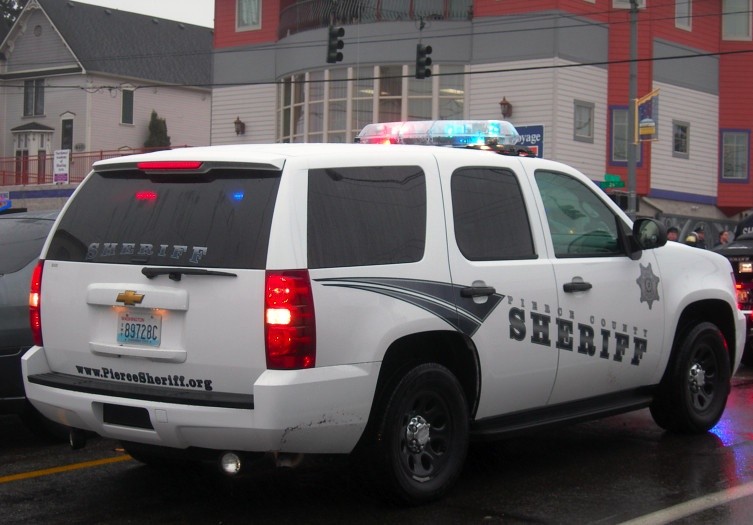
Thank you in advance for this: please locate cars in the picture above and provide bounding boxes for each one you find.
[0,186,102,446]
[712,214,753,332]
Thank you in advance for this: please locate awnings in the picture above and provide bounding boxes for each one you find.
[639,196,727,222]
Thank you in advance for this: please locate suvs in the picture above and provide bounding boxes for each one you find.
[20,118,753,506]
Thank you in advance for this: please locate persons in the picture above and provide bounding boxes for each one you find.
[668,227,729,250]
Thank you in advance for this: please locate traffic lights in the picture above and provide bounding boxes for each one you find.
[415,43,433,81]
[325,26,346,65]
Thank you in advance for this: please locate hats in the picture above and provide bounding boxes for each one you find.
[695,227,705,233]
